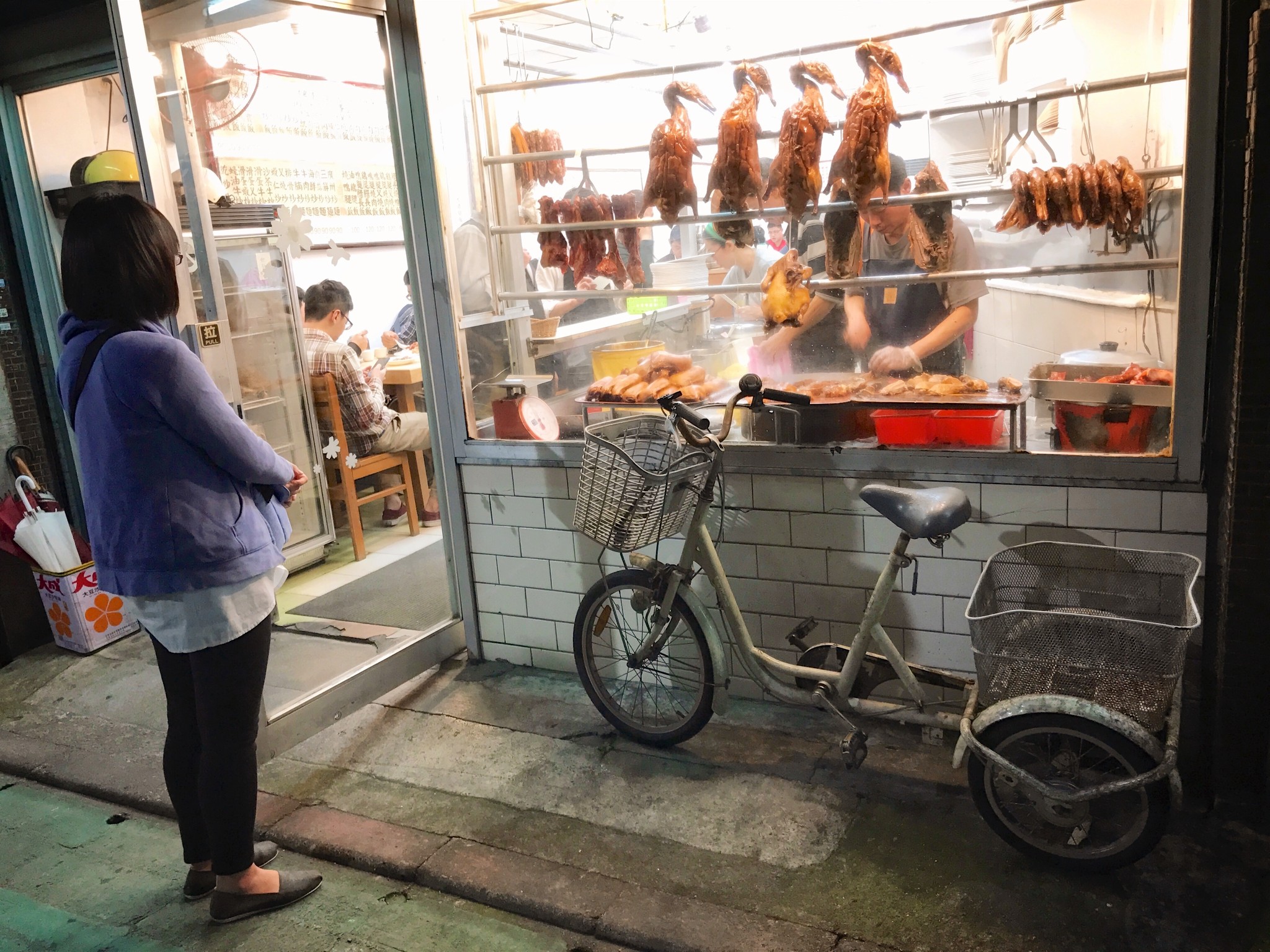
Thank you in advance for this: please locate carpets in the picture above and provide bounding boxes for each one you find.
[285,539,451,631]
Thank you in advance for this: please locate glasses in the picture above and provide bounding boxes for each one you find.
[174,252,182,266]
[406,294,413,301]
[332,309,353,330]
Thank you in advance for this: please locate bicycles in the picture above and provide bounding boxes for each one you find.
[569,376,1203,873]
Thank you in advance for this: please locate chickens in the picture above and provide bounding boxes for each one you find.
[761,249,813,334]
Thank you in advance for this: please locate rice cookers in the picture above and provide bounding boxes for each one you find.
[1040,343,1164,367]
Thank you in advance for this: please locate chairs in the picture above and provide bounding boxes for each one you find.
[311,373,419,561]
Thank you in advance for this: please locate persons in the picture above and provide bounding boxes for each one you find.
[380,267,420,352]
[301,277,454,526]
[291,285,368,358]
[837,150,991,375]
[751,226,774,250]
[700,222,790,320]
[54,191,323,922]
[535,189,635,325]
[738,157,858,372]
[765,222,789,254]
[449,194,532,378]
[607,189,655,313]
[657,224,700,262]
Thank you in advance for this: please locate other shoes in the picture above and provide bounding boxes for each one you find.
[208,868,322,922]
[183,841,280,900]
[423,505,442,527]
[382,503,408,526]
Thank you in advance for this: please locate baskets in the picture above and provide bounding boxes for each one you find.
[964,538,1201,734]
[573,414,714,553]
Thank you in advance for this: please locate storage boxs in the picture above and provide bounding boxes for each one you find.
[31,560,141,657]
[626,296,667,314]
[870,408,1004,445]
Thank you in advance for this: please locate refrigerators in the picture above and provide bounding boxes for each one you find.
[181,204,336,576]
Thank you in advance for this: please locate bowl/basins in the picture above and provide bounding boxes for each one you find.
[680,336,738,366]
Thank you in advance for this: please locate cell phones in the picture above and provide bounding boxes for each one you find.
[368,357,390,376]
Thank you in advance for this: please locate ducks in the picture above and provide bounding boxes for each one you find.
[994,155,1145,236]
[822,40,910,211]
[638,81,717,229]
[762,60,848,222]
[704,62,776,214]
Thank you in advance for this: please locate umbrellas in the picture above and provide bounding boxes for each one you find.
[9,474,82,572]
[0,490,47,568]
[5,443,91,567]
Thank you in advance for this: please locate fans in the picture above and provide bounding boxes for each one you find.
[154,30,260,182]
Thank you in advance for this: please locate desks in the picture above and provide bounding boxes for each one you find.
[362,354,433,517]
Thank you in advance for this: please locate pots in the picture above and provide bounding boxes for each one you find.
[589,341,665,383]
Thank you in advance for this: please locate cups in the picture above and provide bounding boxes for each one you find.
[375,348,387,358]
[249,424,266,441]
[362,349,375,361]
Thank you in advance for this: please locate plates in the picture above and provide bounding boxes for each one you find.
[386,360,413,365]
[394,350,412,356]
[649,252,716,303]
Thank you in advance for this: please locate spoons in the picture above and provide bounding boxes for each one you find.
[395,339,410,349]
